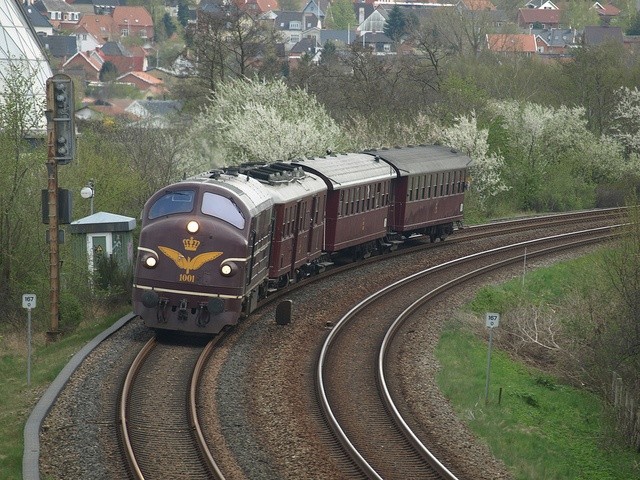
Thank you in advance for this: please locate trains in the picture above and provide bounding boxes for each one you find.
[127,140,473,334]
